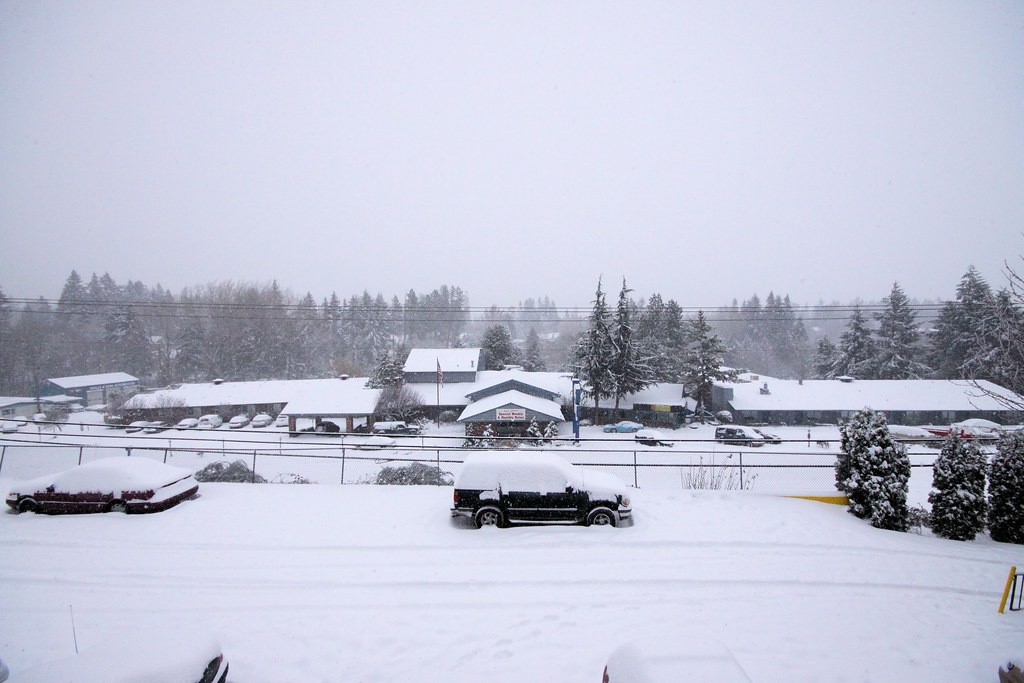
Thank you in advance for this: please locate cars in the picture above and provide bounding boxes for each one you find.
[14,416,28,426]
[228,415,251,429]
[753,427,782,444]
[884,418,1002,447]
[6,458,202,516]
[276,414,289,427]
[143,420,170,433]
[2,420,19,433]
[175,418,199,432]
[252,413,275,429]
[70,404,85,413]
[30,413,47,423]
[126,420,149,433]
[602,421,643,434]
[635,429,675,447]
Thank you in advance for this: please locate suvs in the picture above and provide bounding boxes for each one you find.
[315,421,341,434]
[371,422,421,437]
[450,451,635,529]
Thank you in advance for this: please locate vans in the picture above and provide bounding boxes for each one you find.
[715,426,766,447]
[199,414,223,430]
